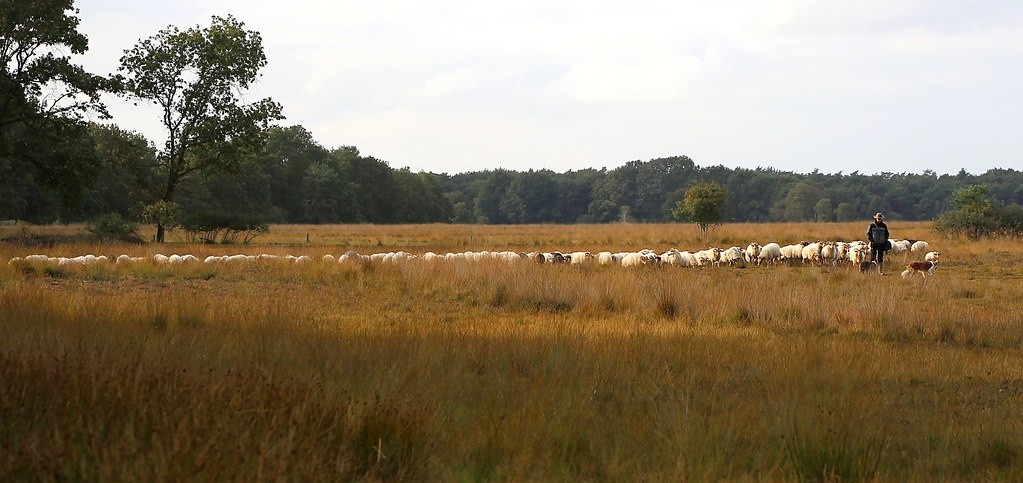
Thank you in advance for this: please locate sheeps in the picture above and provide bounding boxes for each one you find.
[7,237,942,271]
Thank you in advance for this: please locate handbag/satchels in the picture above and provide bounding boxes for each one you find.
[883,240,891,251]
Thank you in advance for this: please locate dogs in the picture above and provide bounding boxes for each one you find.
[901,258,936,279]
[859,258,878,276]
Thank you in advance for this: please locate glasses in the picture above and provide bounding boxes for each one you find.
[878,219,881,220]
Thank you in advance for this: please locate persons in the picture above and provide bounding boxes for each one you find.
[866,213,890,276]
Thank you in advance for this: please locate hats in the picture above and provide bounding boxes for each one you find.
[873,213,884,219]
